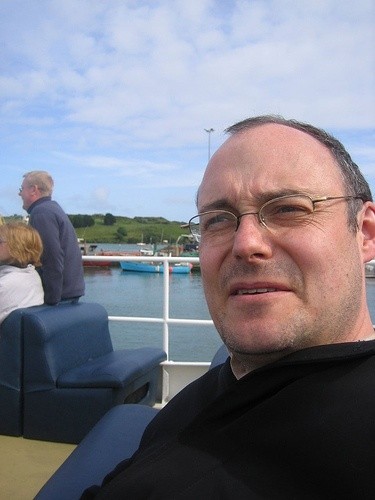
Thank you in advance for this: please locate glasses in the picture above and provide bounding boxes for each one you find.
[177,194,367,248]
[19,185,34,192]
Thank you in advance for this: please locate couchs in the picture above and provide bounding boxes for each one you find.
[0,302,167,446]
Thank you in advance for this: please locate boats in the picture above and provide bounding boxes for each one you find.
[77,230,201,275]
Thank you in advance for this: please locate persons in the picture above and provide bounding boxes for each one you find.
[0,216,45,326]
[19,170,86,307]
[78,115,375,500]
